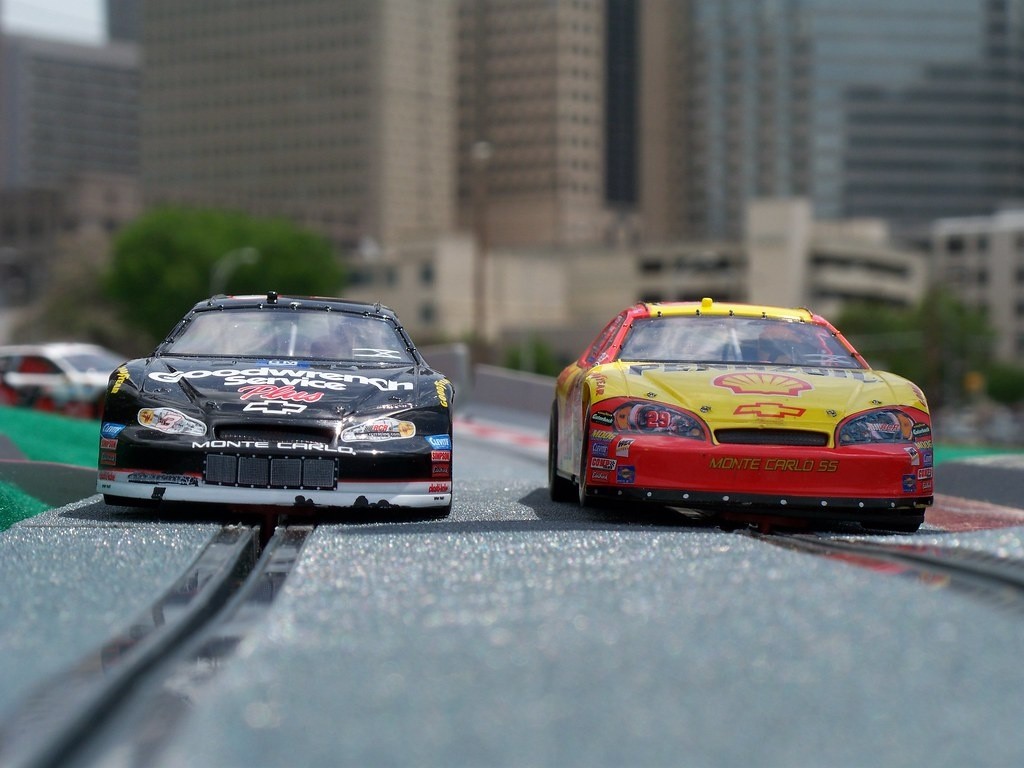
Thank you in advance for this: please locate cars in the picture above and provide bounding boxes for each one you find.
[0,343,132,419]
[549,298,934,533]
[95,291,455,516]
